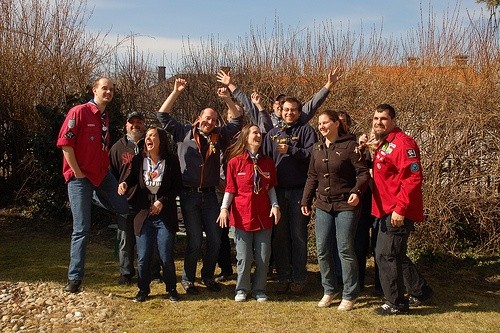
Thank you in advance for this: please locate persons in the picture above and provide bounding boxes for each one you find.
[57,77,129,289]
[215,65,346,280]
[117,126,181,304]
[321,112,371,291]
[358,122,404,299]
[263,97,320,296]
[215,123,281,302]
[206,92,277,284]
[156,78,247,295]
[302,110,372,312]
[356,103,435,314]
[106,111,165,287]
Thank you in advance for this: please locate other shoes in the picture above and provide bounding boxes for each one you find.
[153,274,164,283]
[118,271,136,284]
[202,275,220,291]
[214,274,236,282]
[64,280,82,293]
[181,280,200,296]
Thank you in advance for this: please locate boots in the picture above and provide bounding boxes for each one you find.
[289,276,309,294]
[269,278,293,293]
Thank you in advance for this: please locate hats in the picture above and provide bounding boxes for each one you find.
[127,112,145,122]
[275,93,288,101]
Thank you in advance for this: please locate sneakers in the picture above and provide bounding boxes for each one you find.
[337,294,359,311]
[168,291,179,303]
[132,290,150,303]
[251,292,269,302]
[318,291,341,308]
[374,304,400,315]
[407,287,432,306]
[235,289,249,302]
